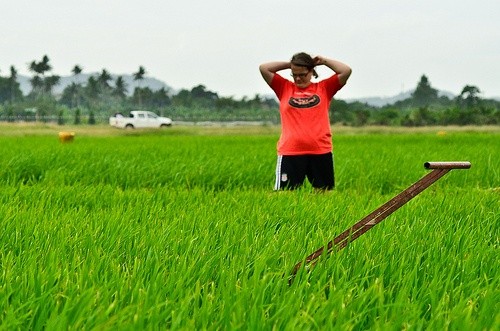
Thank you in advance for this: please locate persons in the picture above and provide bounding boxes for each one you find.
[259,52,353,193]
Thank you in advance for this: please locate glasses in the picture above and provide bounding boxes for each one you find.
[290,71,310,78]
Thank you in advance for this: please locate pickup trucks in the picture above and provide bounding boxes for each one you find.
[108,111,172,130]
